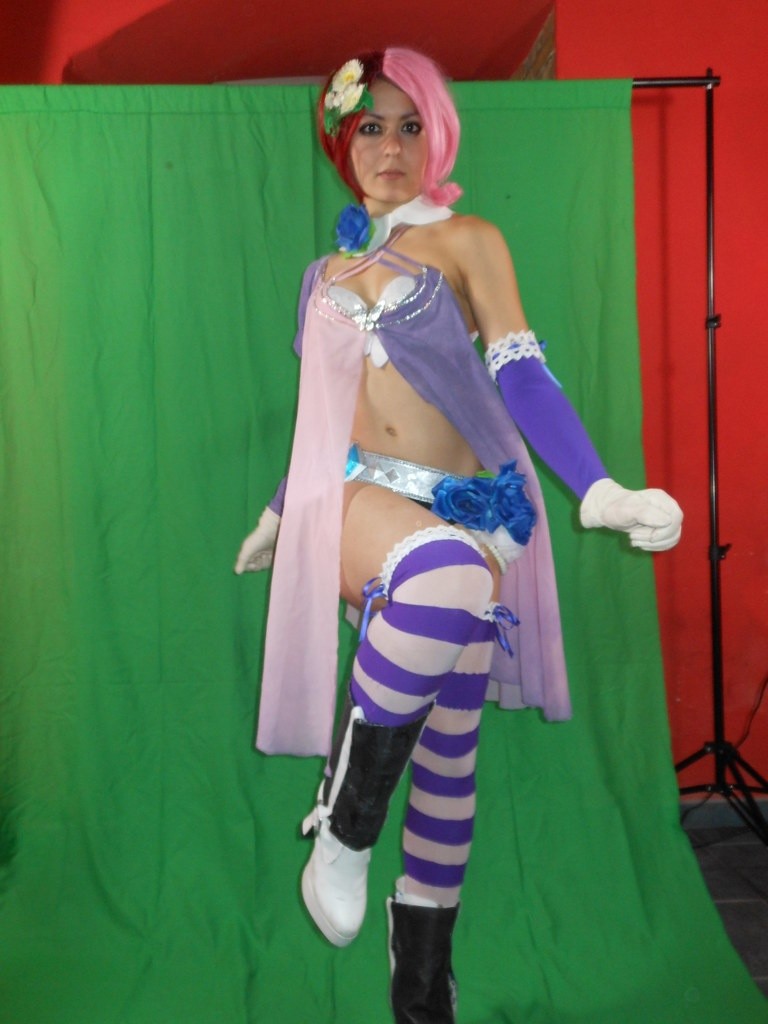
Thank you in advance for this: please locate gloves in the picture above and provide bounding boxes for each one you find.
[234,507,281,574]
[579,476,684,552]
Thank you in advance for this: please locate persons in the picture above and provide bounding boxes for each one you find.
[233,50,685,1023]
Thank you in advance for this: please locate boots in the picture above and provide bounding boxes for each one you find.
[300,681,436,947]
[383,895,459,1024]
